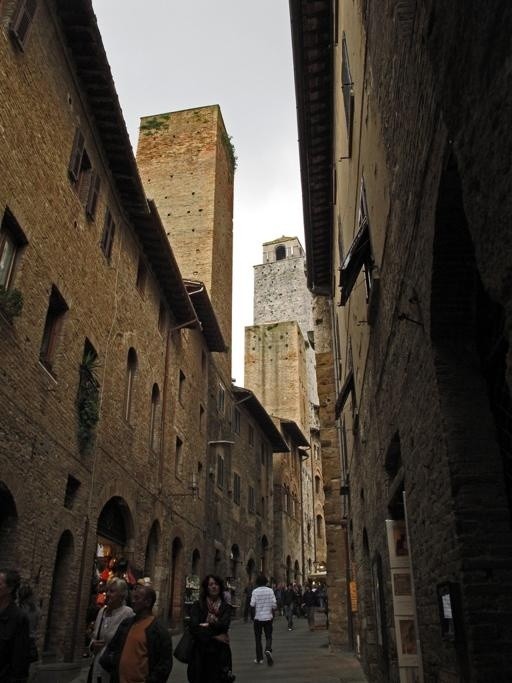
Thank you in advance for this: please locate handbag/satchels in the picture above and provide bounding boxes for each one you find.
[86,655,95,683]
[172,624,200,664]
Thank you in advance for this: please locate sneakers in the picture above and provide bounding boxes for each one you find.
[253,657,265,664]
[265,650,274,665]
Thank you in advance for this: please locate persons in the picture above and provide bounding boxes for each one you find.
[100,586,171,683]
[14,578,40,627]
[220,581,331,633]
[2,561,33,682]
[86,575,135,682]
[188,575,236,682]
[248,575,278,668]
